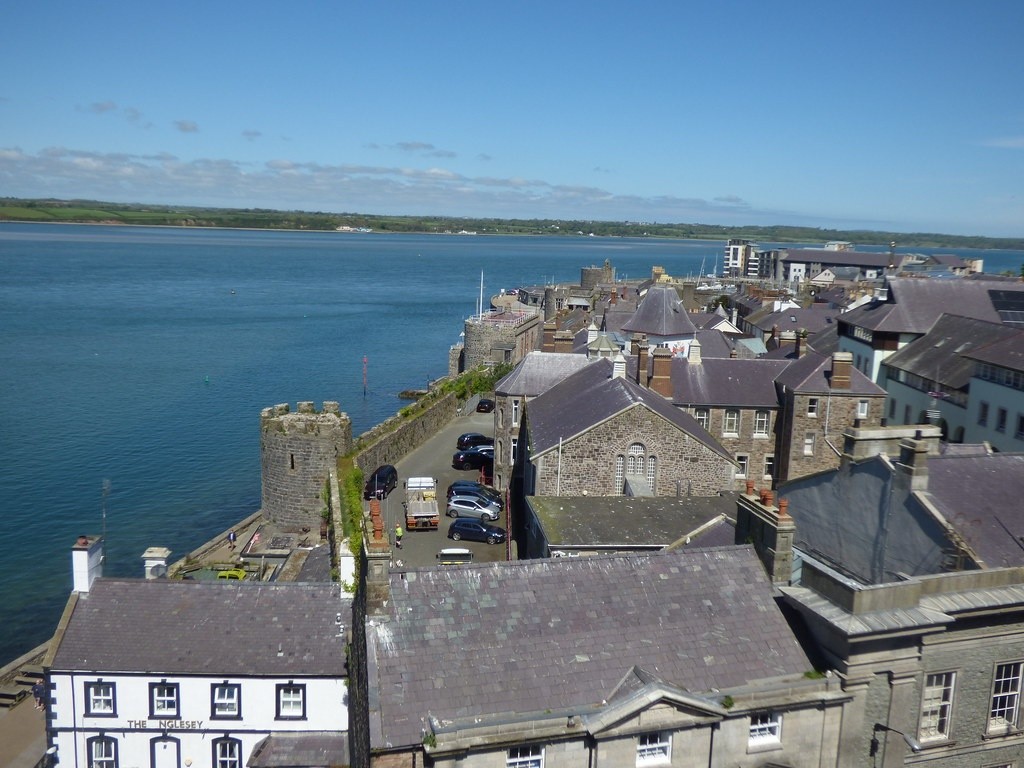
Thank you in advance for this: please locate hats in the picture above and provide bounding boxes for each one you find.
[397,524,399,526]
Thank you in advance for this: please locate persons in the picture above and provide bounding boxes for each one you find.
[228,530,236,551]
[31,679,46,709]
[395,524,403,549]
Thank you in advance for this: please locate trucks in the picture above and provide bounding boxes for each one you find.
[402,477,439,530]
[436,548,474,565]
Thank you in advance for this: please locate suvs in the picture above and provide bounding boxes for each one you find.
[457,432,494,448]
[453,449,493,471]
[363,465,398,500]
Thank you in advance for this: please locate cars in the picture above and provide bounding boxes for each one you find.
[477,398,494,413]
[448,517,508,545]
[445,481,504,512]
[446,494,501,522]
[470,444,494,459]
